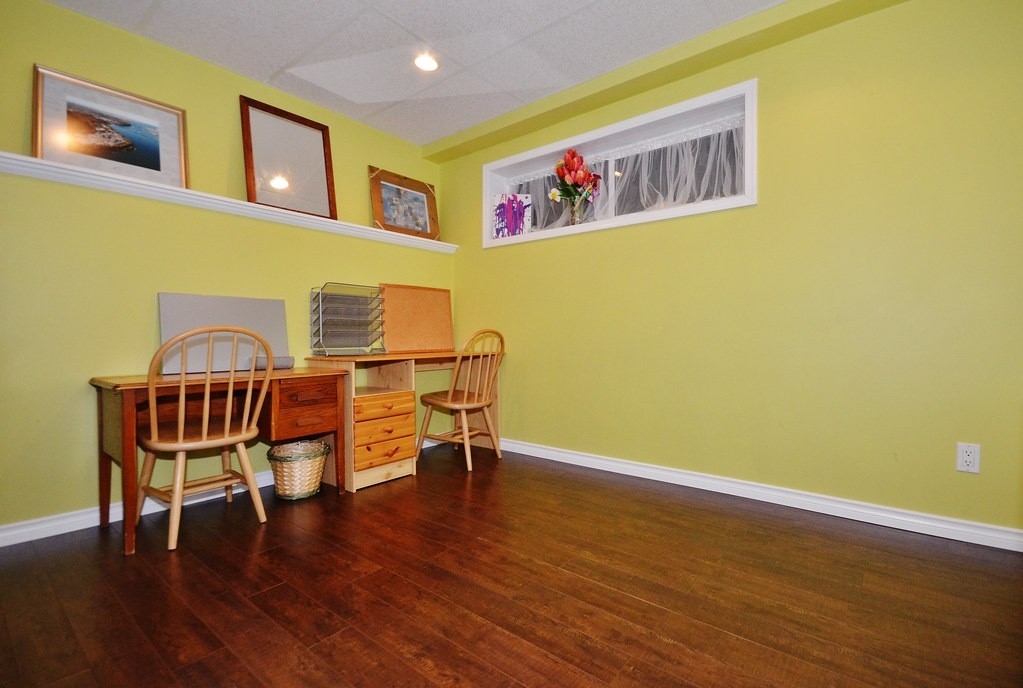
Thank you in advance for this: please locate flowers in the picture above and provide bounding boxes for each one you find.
[547,148,602,210]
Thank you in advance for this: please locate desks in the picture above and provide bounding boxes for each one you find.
[88,366,351,557]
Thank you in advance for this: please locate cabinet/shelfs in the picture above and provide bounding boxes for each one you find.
[303,352,506,496]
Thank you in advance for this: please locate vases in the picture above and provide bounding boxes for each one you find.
[568,195,583,226]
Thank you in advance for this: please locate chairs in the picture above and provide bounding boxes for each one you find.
[136,325,274,552]
[415,329,508,472]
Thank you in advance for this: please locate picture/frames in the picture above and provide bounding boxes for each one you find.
[368,164,441,242]
[31,62,190,189]
[239,94,338,221]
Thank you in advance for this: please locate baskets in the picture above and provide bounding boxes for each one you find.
[267,439,331,500]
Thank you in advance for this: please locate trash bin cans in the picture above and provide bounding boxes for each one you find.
[266,439,330,501]
